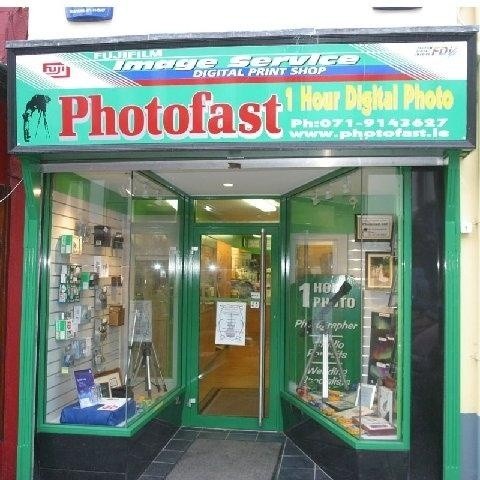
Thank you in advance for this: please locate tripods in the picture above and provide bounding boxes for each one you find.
[133,350,160,397]
[297,333,349,403]
